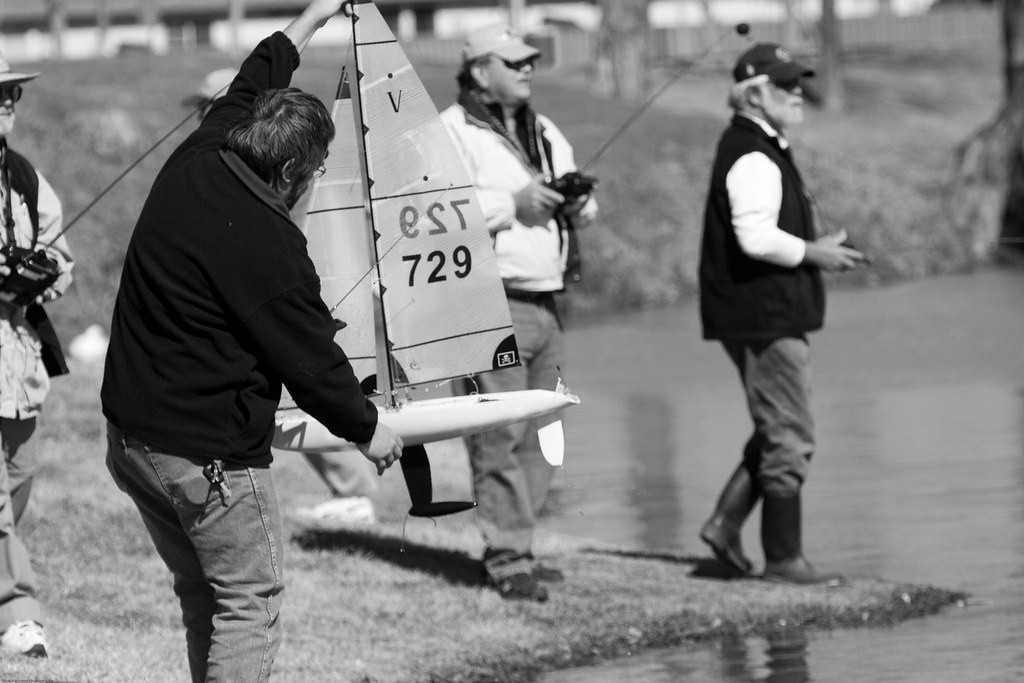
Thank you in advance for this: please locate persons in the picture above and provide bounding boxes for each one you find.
[436,22,599,600]
[700,44,864,583]
[1,61,74,658]
[100,0,404,682]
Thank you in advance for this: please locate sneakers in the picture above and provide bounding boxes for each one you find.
[0,620,51,657]
[482,547,564,603]
[294,495,376,528]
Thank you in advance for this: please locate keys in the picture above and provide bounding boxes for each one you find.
[203,459,232,498]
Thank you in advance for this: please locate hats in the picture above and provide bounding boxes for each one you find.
[732,42,816,81]
[183,66,239,101]
[0,59,41,83]
[459,21,543,64]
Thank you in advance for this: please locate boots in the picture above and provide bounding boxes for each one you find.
[700,458,761,573]
[761,489,846,587]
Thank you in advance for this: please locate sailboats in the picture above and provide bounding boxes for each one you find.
[268,0,578,474]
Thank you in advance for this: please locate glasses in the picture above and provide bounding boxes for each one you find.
[306,161,327,179]
[0,83,23,104]
